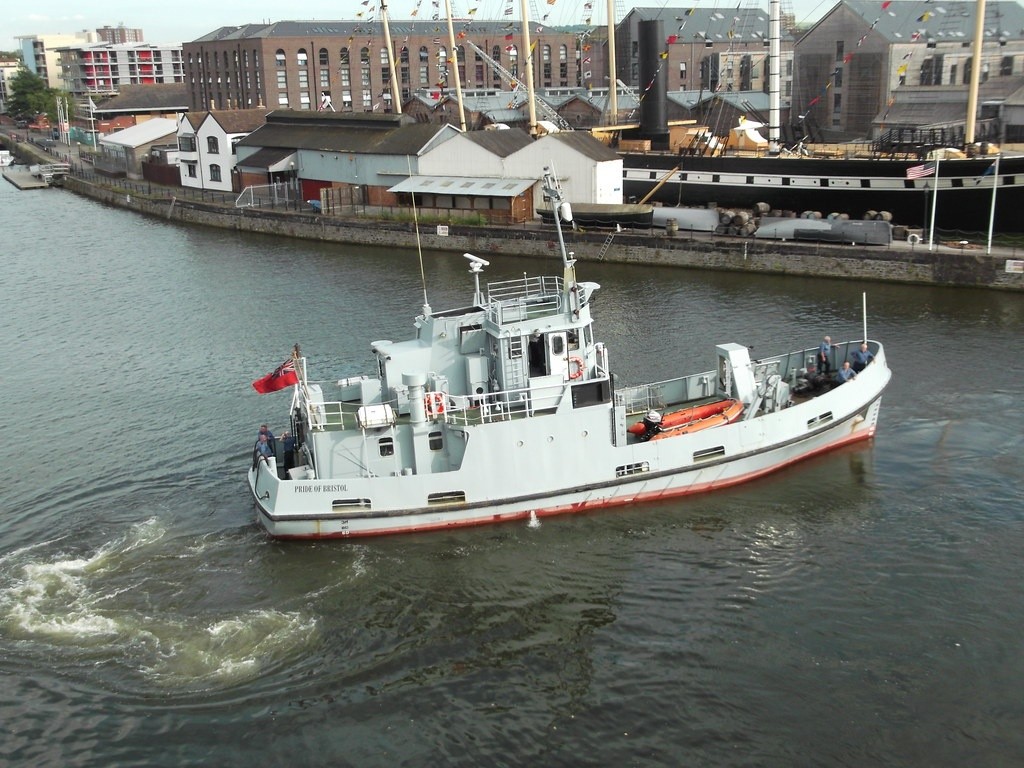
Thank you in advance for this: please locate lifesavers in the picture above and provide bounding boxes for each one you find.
[423,393,445,415]
[567,356,586,380]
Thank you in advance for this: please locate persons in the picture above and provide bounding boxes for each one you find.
[279,430,295,480]
[830,362,856,390]
[817,335,840,376]
[850,343,876,374]
[256,425,276,458]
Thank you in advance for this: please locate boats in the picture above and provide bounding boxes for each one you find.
[248,168,890,543]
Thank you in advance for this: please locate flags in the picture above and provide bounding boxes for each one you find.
[505,44,513,52]
[582,44,591,50]
[253,357,299,393]
[976,159,996,185]
[505,6,513,16]
[584,70,591,79]
[582,56,591,63]
[906,160,936,179]
[506,33,513,40]
